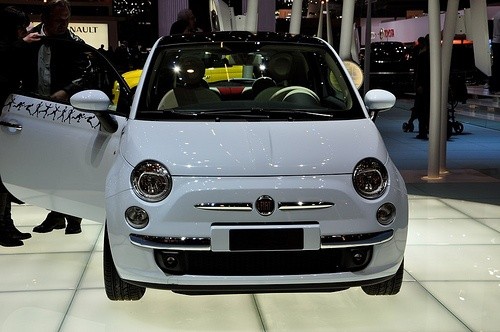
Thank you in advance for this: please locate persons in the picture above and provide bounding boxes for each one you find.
[0,5,42,247]
[413,39,430,140]
[21,0,97,236]
[170,9,196,36]
[98,44,106,51]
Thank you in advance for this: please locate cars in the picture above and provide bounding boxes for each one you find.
[360,40,415,69]
[0,33,408,302]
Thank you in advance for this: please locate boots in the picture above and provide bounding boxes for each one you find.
[0,224,24,247]
[6,219,31,240]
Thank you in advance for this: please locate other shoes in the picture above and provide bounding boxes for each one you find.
[33,219,65,233]
[64,223,81,234]
[416,135,427,139]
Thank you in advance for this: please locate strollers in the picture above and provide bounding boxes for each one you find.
[402,89,464,138]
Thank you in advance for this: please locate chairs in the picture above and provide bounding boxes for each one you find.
[253,84,293,102]
[157,86,221,111]
[177,56,209,90]
[267,54,298,88]
